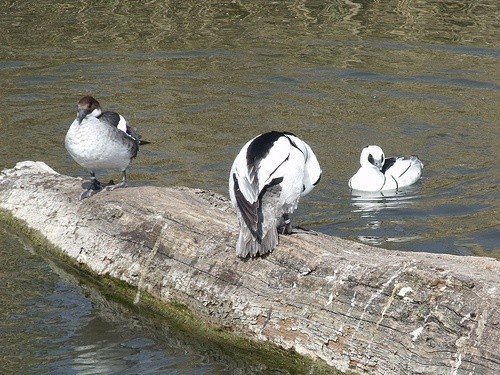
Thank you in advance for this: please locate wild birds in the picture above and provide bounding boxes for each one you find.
[71,315,140,375]
[351,189,427,246]
[228,131,323,258]
[348,145,425,192]
[64,95,155,201]
